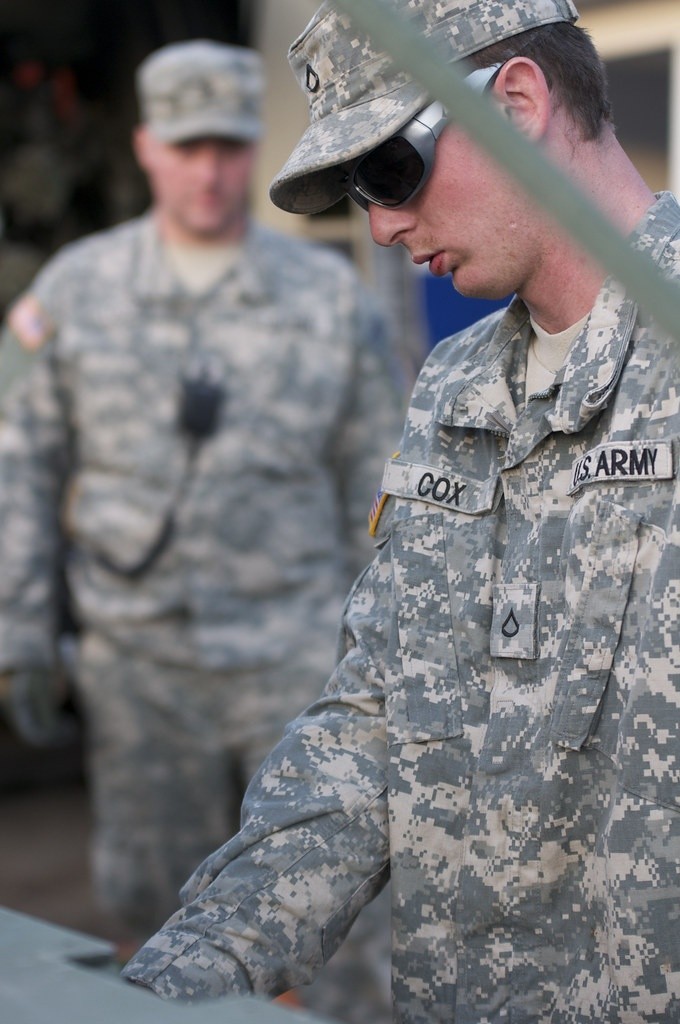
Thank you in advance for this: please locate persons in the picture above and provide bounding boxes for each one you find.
[114,0,680,1023]
[1,43,415,964]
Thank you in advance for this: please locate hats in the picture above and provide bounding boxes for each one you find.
[271,3,579,207]
[138,41,272,145]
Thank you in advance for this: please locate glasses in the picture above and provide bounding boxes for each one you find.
[342,64,502,210]
